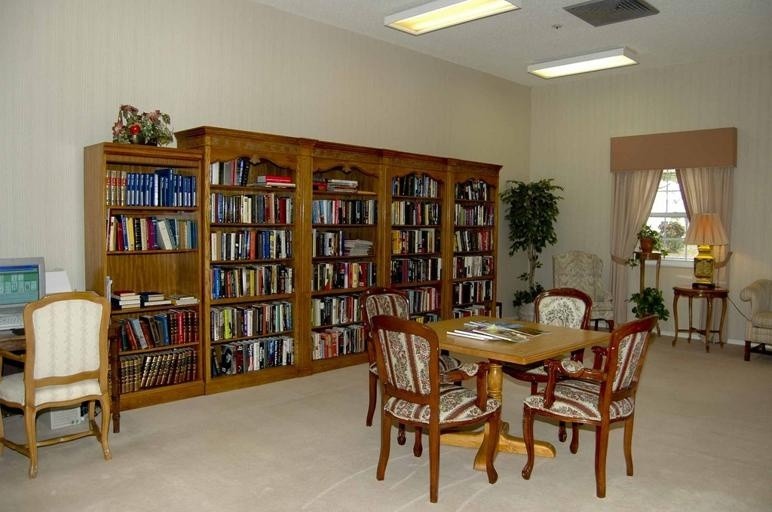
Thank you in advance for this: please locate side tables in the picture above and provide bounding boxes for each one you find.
[671,285,730,353]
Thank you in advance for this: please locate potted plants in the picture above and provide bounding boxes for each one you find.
[498,178,565,322]
[625,224,668,269]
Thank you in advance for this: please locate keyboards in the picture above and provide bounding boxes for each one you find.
[0,315,24,330]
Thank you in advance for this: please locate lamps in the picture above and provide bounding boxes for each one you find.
[383,0,522,37]
[527,46,640,81]
[683,212,728,290]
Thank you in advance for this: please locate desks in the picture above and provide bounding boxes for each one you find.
[423,315,611,473]
[0,329,27,375]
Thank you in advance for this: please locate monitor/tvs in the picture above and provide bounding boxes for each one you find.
[0,256,46,309]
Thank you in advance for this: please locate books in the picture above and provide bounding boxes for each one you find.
[107,346,199,397]
[452,254,495,279]
[312,176,372,192]
[210,192,295,225]
[210,263,295,301]
[210,300,293,342]
[209,156,297,189]
[390,175,440,198]
[389,227,442,255]
[108,310,201,354]
[106,213,199,252]
[309,293,362,328]
[390,200,441,226]
[451,280,493,305]
[446,319,552,343]
[311,260,378,291]
[105,168,198,208]
[210,228,294,262]
[312,198,377,226]
[310,323,366,361]
[403,286,441,323]
[310,228,375,258]
[451,304,494,319]
[453,181,494,253]
[106,274,199,310]
[389,256,443,286]
[210,334,295,378]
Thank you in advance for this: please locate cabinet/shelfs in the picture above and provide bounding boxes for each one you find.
[173,125,503,395]
[83,142,204,414]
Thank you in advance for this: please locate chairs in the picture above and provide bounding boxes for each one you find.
[488,287,592,442]
[359,285,463,446]
[0,290,112,479]
[552,250,616,333]
[369,315,503,504]
[522,314,659,498]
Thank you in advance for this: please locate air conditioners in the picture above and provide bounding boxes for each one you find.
[562,0,660,28]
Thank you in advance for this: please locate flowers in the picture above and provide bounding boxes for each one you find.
[112,104,174,147]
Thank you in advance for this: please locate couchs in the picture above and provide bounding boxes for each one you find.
[739,278,772,362]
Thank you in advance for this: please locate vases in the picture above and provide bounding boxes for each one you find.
[127,135,146,145]
